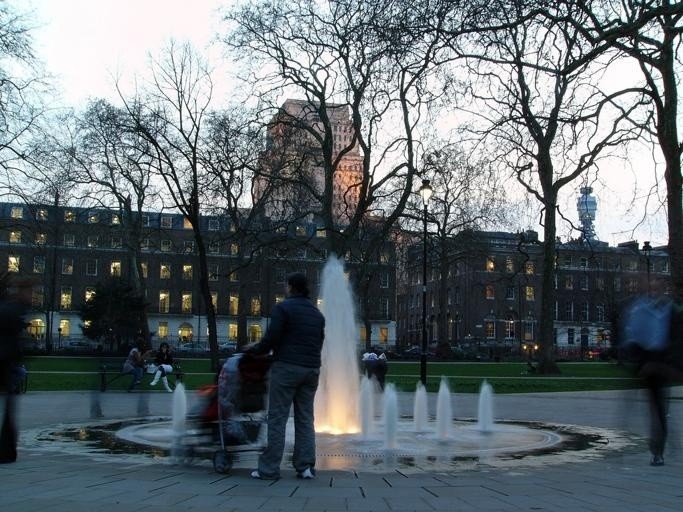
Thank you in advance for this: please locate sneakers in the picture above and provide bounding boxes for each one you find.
[294,466,315,480]
[249,468,280,482]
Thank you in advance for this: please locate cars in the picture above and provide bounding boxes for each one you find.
[377,344,603,362]
[21,334,240,357]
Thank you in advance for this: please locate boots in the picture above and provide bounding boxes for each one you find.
[149,369,162,386]
[160,375,173,393]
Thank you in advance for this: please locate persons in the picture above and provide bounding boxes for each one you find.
[121,336,155,395]
[612,265,683,468]
[148,341,175,393]
[240,270,325,481]
[0,278,25,464]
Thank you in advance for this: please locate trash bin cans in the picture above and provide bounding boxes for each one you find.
[363,360,388,393]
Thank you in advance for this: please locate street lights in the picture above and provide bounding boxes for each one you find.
[416,175,435,386]
[56,327,62,349]
[578,318,583,348]
[641,240,652,298]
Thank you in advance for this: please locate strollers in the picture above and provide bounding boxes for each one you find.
[160,348,268,476]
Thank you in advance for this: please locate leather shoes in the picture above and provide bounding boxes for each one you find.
[649,454,663,467]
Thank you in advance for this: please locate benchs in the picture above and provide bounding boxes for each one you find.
[100,358,184,391]
